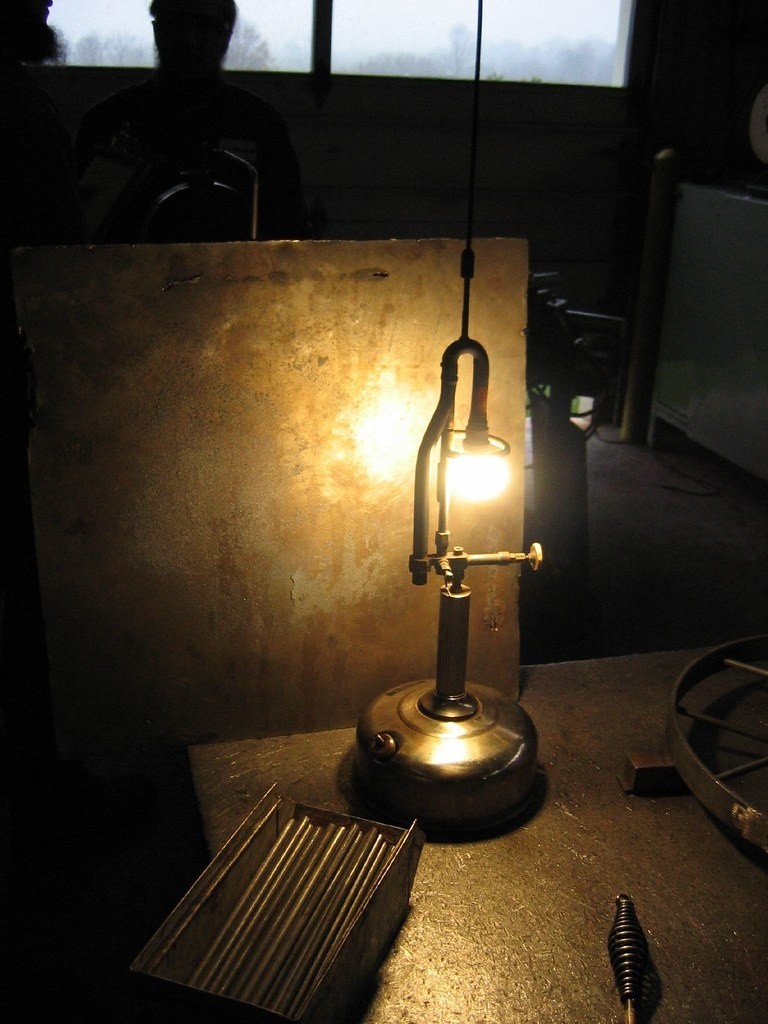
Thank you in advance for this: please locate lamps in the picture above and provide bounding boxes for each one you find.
[353,0,546,844]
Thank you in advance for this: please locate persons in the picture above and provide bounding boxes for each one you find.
[64,0,312,244]
